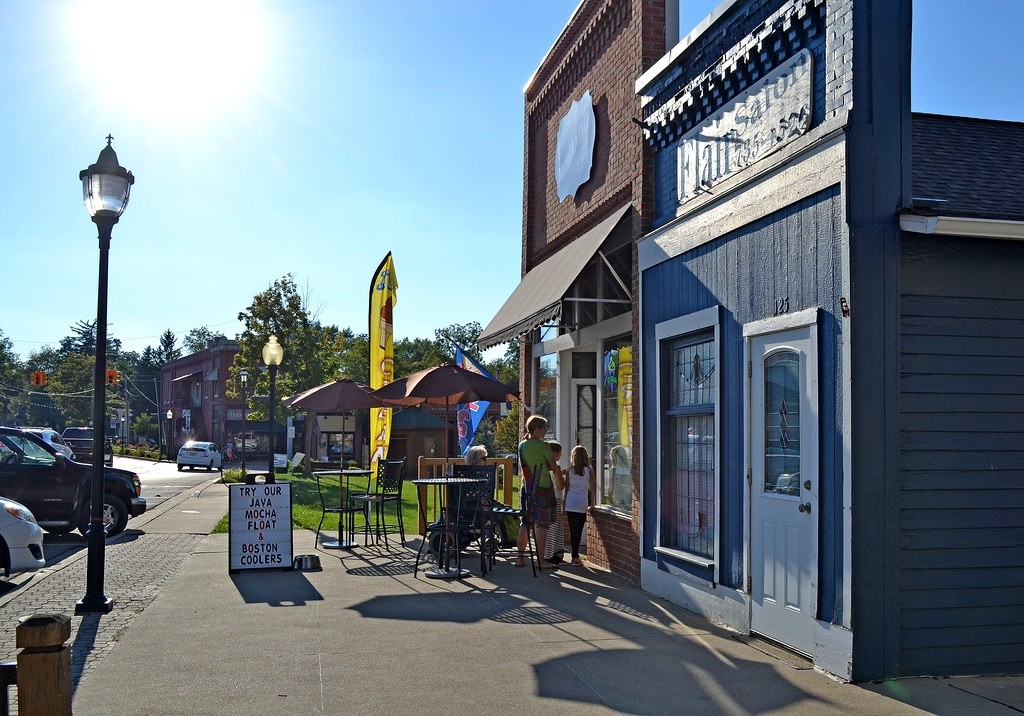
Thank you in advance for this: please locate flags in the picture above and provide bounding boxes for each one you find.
[455,347,499,457]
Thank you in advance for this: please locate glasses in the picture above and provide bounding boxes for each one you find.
[480,457,486,461]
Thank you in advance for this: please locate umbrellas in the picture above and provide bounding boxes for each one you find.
[281,377,404,520]
[369,362,521,544]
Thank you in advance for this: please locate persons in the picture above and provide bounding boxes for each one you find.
[544,443,566,565]
[565,445,596,568]
[516,416,556,568]
[609,447,633,508]
[464,444,521,549]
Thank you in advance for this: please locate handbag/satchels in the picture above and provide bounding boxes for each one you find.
[525,487,558,526]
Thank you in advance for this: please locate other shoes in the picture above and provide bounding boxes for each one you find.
[559,559,569,565]
[570,560,584,566]
[516,561,525,566]
[536,564,555,569]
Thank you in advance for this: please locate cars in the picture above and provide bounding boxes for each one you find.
[177,442,223,471]
[235,433,260,448]
[0,496,46,578]
[61,427,113,467]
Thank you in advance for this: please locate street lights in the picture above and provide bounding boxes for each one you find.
[262,334,283,483]
[75,134,135,612]
[239,368,249,470]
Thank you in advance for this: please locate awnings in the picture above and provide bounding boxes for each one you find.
[476,200,633,354]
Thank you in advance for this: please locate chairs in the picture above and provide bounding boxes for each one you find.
[448,461,544,577]
[347,457,408,551]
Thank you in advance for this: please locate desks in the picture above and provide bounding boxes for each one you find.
[312,470,373,549]
[410,478,490,578]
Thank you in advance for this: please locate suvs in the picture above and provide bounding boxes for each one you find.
[0,427,74,463]
[0,427,146,538]
